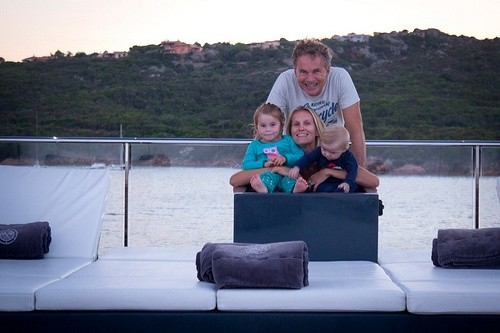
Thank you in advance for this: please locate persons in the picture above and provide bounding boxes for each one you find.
[241,102,308,193]
[289,124,358,193]
[230,106,380,194]
[266,39,366,170]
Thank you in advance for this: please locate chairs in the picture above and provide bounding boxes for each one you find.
[1,167,500,312]
